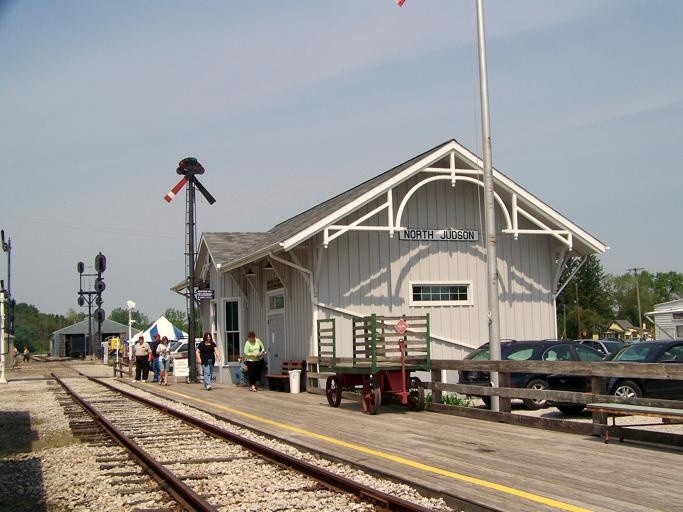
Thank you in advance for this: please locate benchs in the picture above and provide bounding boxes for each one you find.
[264,360,306,392]
[585,402,682,443]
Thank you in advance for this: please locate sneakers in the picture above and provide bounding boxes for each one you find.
[249,387,257,392]
[204,385,215,390]
[131,380,167,386]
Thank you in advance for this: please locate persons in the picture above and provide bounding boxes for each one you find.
[195,331,222,391]
[236,348,269,387]
[152,333,163,382]
[23,346,30,362]
[132,336,153,383]
[156,336,172,386]
[12,345,18,365]
[243,331,267,392]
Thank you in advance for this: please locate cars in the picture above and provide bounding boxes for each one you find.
[170,337,203,370]
[454,337,683,416]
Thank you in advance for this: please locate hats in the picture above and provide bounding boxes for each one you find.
[153,333,160,337]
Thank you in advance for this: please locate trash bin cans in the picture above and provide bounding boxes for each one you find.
[96,347,103,359]
[287,369,301,394]
